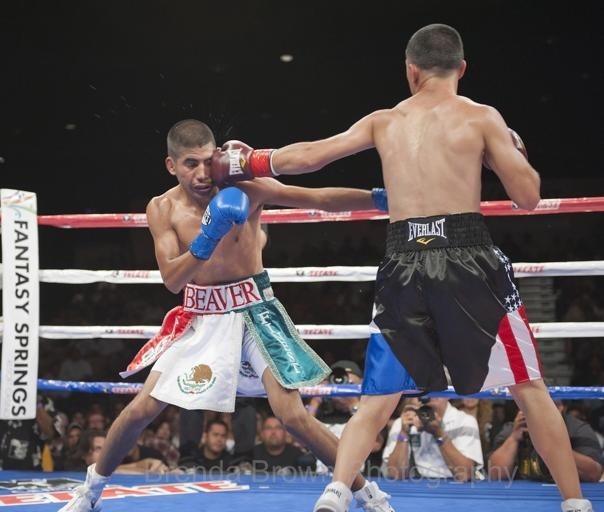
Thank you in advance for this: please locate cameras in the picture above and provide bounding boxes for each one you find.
[328,367,348,385]
[416,396,434,421]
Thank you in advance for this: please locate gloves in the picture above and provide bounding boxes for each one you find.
[189,187,250,261]
[482,127,529,171]
[371,187,389,212]
[210,140,280,188]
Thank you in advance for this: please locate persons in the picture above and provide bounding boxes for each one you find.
[53,119,399,510]
[0,335,603,485]
[210,22,596,512]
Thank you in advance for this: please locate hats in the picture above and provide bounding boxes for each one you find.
[331,360,363,378]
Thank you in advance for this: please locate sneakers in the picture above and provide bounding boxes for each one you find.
[56,463,110,512]
[312,481,353,511]
[354,480,396,512]
[560,498,595,512]
